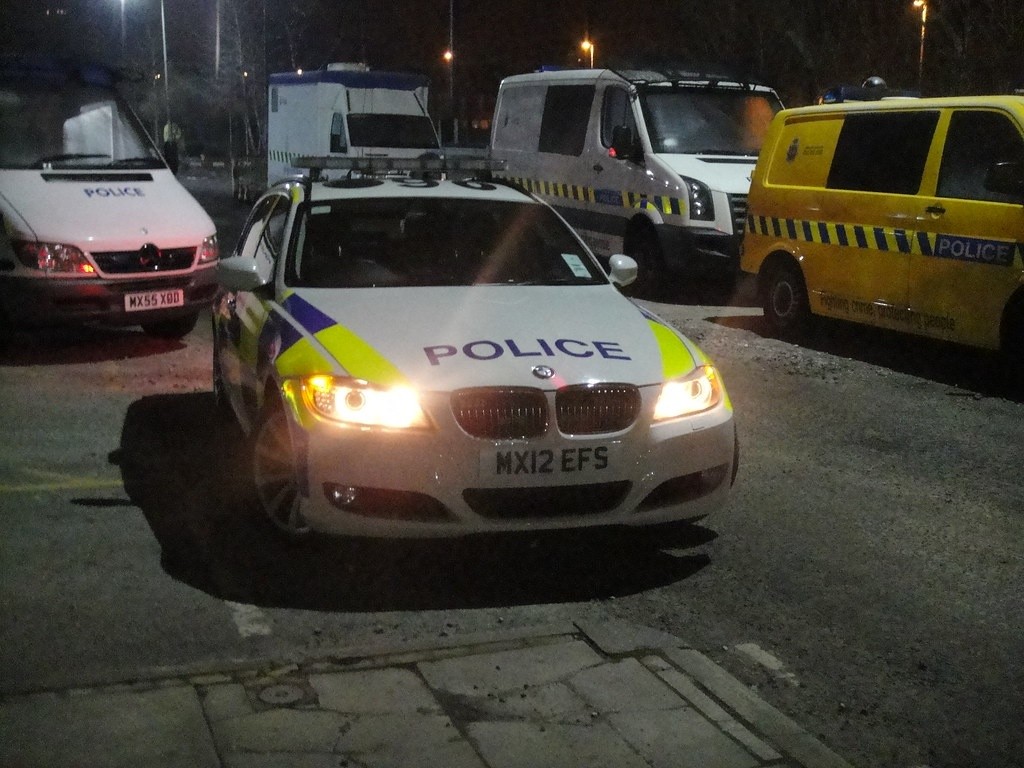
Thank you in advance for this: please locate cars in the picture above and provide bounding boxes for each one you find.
[200,154,740,547]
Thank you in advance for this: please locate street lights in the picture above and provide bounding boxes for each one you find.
[913,0,928,97]
[580,41,594,68]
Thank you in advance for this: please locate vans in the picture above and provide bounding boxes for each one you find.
[262,57,448,194]
[0,41,223,343]
[739,94,1024,357]
[488,64,791,299]
